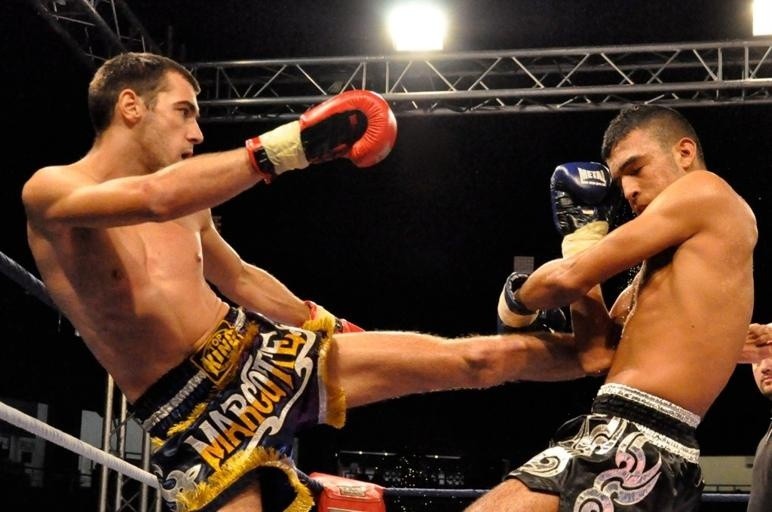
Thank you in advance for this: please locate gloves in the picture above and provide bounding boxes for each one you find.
[245,89,398,183]
[302,300,364,334]
[549,162,611,257]
[496,270,566,334]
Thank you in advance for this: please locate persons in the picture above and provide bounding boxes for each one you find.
[460,104,759,512]
[21,51,772,512]
[747,324,772,512]
